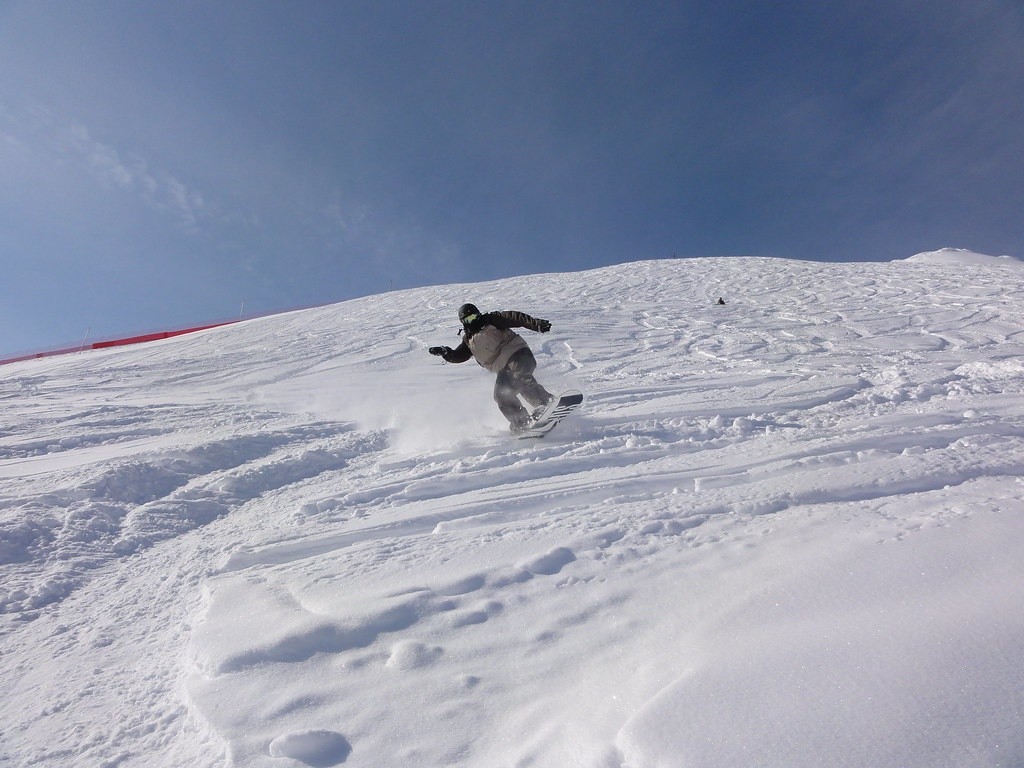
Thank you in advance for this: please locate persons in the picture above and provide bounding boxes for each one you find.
[429,304,558,433]
[718,297,725,304]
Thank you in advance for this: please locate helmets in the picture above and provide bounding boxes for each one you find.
[458,303,479,320]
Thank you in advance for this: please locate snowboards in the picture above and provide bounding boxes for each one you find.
[517,389,585,440]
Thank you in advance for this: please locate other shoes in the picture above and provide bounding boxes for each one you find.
[508,413,531,431]
[530,395,556,420]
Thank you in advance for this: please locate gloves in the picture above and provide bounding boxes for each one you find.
[541,320,552,333]
[429,347,447,356]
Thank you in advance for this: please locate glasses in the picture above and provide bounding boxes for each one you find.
[461,311,481,325]
